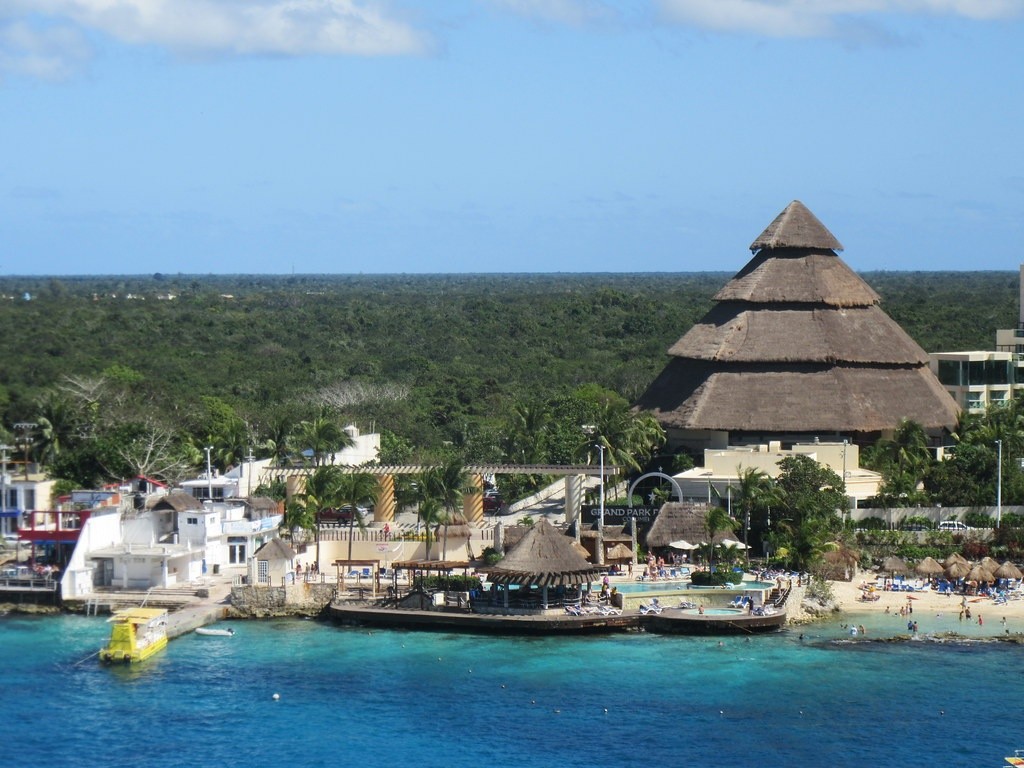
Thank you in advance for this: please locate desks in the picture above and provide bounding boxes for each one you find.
[3,566,29,577]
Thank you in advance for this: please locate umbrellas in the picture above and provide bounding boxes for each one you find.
[571,541,591,558]
[607,543,634,572]
[877,554,1022,591]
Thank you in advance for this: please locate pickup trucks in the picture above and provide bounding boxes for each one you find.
[313,507,354,525]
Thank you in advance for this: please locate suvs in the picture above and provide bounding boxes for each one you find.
[937,520,967,530]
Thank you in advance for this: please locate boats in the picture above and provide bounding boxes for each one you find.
[195,627,236,636]
[99,607,168,664]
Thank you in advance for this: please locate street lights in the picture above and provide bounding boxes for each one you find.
[594,444,606,526]
[204,446,214,499]
[995,440,1002,529]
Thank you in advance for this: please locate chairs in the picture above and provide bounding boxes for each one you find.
[860,584,880,603]
[348,568,402,579]
[995,597,1002,604]
[677,595,695,608]
[639,598,663,615]
[566,605,621,615]
[642,568,691,581]
[763,569,799,581]
[753,604,777,617]
[727,595,750,607]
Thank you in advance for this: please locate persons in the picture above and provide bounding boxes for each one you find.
[558,584,578,599]
[490,583,494,596]
[644,551,664,581]
[602,564,620,599]
[670,551,708,572]
[628,560,634,577]
[698,604,705,615]
[999,616,1010,635]
[850,624,865,635]
[976,615,982,625]
[748,596,754,616]
[296,561,319,582]
[858,579,1008,632]
[756,567,790,592]
[37,563,59,580]
[383,523,389,534]
[456,593,462,607]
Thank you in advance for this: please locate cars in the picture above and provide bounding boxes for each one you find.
[338,504,367,519]
[898,525,930,531]
[482,491,504,511]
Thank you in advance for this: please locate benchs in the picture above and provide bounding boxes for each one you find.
[472,588,588,608]
